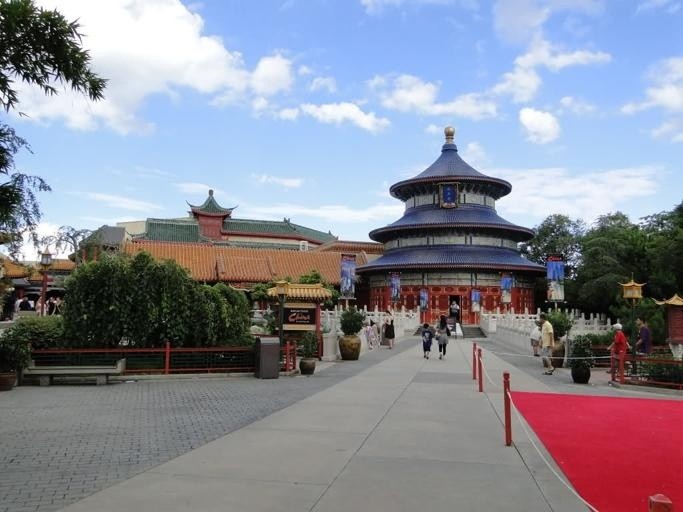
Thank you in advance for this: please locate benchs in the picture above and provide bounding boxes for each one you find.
[22,357,128,386]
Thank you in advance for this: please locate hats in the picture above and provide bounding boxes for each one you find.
[612,323,622,330]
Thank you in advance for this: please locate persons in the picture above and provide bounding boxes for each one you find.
[537,312,556,376]
[368,318,378,345]
[12,291,62,318]
[632,315,653,375]
[383,310,394,351]
[604,321,634,373]
[449,300,460,325]
[529,324,542,357]
[433,314,451,360]
[419,323,434,359]
[362,321,380,350]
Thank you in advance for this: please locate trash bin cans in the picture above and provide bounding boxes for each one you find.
[382,324,395,345]
[254,337,280,379]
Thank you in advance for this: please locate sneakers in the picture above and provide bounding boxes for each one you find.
[542,367,555,375]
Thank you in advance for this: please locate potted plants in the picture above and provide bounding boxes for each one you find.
[0,325,30,391]
[535,308,574,368]
[299,328,318,375]
[570,334,594,384]
[338,306,366,361]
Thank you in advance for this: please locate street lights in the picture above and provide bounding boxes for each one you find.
[617,272,646,378]
[38,245,53,318]
